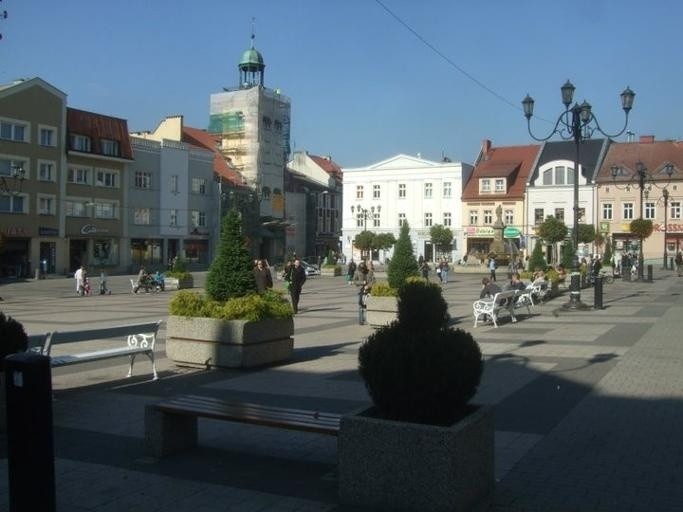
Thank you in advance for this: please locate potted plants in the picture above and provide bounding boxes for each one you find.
[320,250,341,276]
[163,204,294,368]
[163,252,194,290]
[367,216,432,327]
[337,280,491,509]
[596,238,613,276]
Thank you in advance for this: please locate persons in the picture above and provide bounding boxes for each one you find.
[504,260,526,304]
[675,252,683,277]
[530,266,549,291]
[74,265,87,296]
[285,261,292,274]
[285,259,306,315]
[482,278,497,299]
[132,266,164,295]
[253,259,273,294]
[463,253,467,263]
[317,252,376,326]
[480,254,484,264]
[100,268,106,294]
[554,264,567,283]
[621,252,639,275]
[487,255,497,282]
[571,254,601,286]
[418,255,451,284]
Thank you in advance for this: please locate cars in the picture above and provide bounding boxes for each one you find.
[300,261,319,276]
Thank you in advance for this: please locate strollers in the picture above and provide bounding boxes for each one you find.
[83,281,94,297]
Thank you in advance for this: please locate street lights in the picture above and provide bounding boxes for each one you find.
[661,186,671,270]
[145,235,157,272]
[608,158,676,282]
[0,161,26,304]
[288,248,296,264]
[350,204,382,264]
[520,80,639,314]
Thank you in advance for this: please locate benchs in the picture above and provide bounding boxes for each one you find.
[139,387,365,477]
[0,331,50,385]
[129,278,163,294]
[472,269,567,328]
[47,313,162,401]
